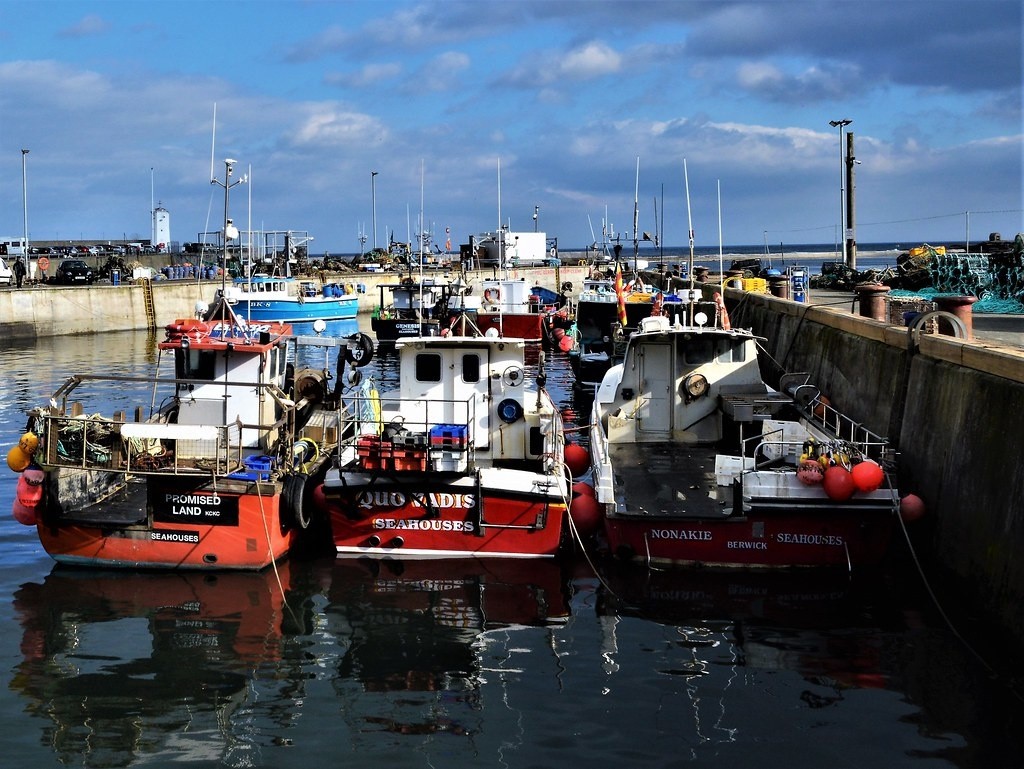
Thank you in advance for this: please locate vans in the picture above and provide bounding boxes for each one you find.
[0,238,29,256]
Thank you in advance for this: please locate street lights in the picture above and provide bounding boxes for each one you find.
[829,119,853,263]
[22,148,30,279]
[371,172,377,248]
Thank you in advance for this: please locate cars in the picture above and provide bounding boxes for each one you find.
[55,259,94,285]
[28,243,157,258]
[0,256,13,285]
[11,158,313,571]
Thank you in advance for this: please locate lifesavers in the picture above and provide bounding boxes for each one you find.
[623,280,636,291]
[485,288,500,304]
[38,257,49,270]
[650,292,664,317]
[165,324,210,343]
[713,292,731,331]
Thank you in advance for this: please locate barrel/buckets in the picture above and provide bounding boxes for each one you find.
[155,265,217,282]
[322,284,343,298]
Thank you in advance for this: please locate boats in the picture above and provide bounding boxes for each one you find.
[365,161,469,334]
[583,154,904,596]
[10,545,300,760]
[208,162,355,323]
[317,543,579,742]
[464,158,568,347]
[557,154,694,388]
[327,157,574,565]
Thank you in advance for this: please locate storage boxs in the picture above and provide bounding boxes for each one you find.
[356,422,467,474]
[228,454,276,480]
